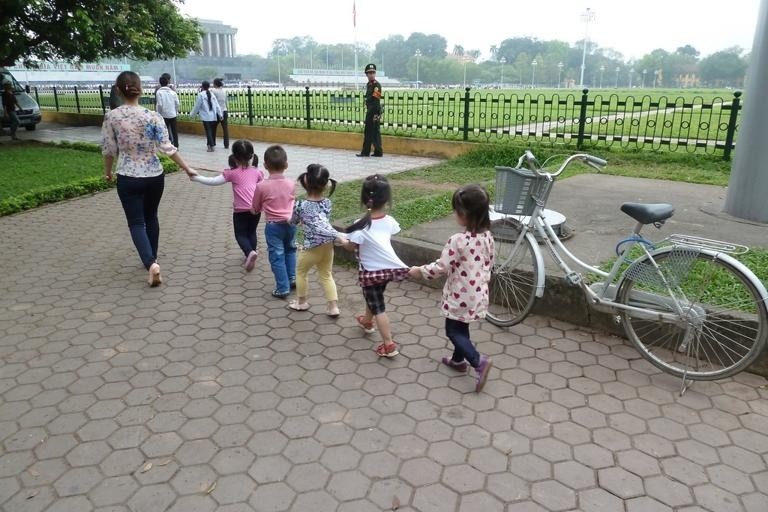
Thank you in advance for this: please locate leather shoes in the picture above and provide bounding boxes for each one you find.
[324,306,341,317]
[371,151,384,157]
[356,152,369,157]
[290,299,310,311]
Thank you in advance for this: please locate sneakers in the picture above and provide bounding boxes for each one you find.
[245,250,259,271]
[474,354,492,393]
[206,144,215,152]
[224,145,229,149]
[442,355,467,372]
[10,135,21,140]
[271,278,296,297]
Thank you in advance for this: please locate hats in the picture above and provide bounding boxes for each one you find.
[364,63,377,73]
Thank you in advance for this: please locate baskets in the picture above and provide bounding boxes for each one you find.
[493,165,553,216]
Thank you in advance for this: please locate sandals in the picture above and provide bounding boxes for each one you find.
[376,342,399,358]
[356,314,374,334]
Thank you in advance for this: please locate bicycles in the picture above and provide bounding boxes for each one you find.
[487,149,767,398]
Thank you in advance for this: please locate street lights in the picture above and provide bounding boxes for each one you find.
[557,61,563,89]
[500,56,507,90]
[578,7,591,87]
[276,44,385,89]
[530,59,537,90]
[460,53,469,90]
[414,49,422,90]
[598,65,659,89]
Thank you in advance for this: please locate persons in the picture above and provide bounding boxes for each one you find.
[210,77,229,149]
[99,67,200,287]
[284,160,340,319]
[185,136,265,271]
[1,79,25,141]
[402,183,495,395]
[331,174,412,358]
[155,76,181,150]
[249,143,297,299]
[154,73,176,145]
[356,64,384,158]
[189,80,224,152]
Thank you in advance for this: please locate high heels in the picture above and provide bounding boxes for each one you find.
[147,263,162,288]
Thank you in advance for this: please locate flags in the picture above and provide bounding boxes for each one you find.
[352,2,357,30]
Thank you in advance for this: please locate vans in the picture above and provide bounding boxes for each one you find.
[0,66,43,131]
